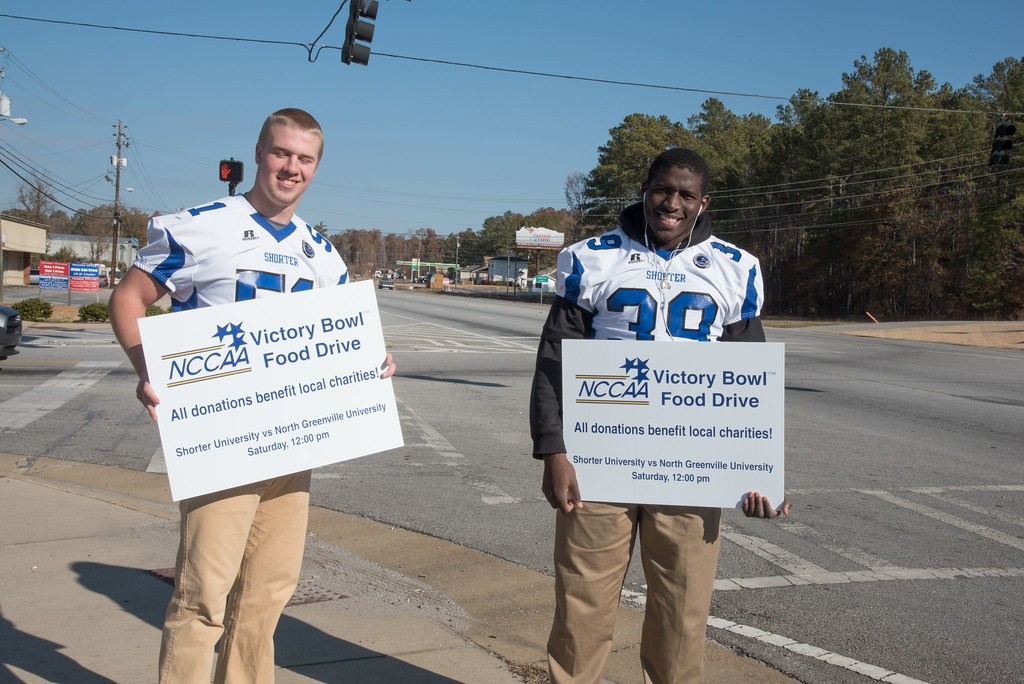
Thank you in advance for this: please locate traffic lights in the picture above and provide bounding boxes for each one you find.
[340,0,379,67]
[218,159,244,182]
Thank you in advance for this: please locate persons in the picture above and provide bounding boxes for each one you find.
[109,108,397,684]
[529,147,792,684]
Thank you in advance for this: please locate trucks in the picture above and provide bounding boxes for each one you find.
[87,262,123,285]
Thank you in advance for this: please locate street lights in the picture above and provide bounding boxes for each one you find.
[0,117,28,302]
[103,174,135,289]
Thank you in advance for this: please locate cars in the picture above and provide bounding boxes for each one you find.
[373,268,405,279]
[413,271,454,284]
[29,269,39,285]
[98,274,109,288]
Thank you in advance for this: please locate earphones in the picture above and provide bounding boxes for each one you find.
[643,191,646,202]
[698,204,703,216]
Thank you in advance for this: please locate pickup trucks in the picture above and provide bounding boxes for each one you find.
[378,278,395,290]
